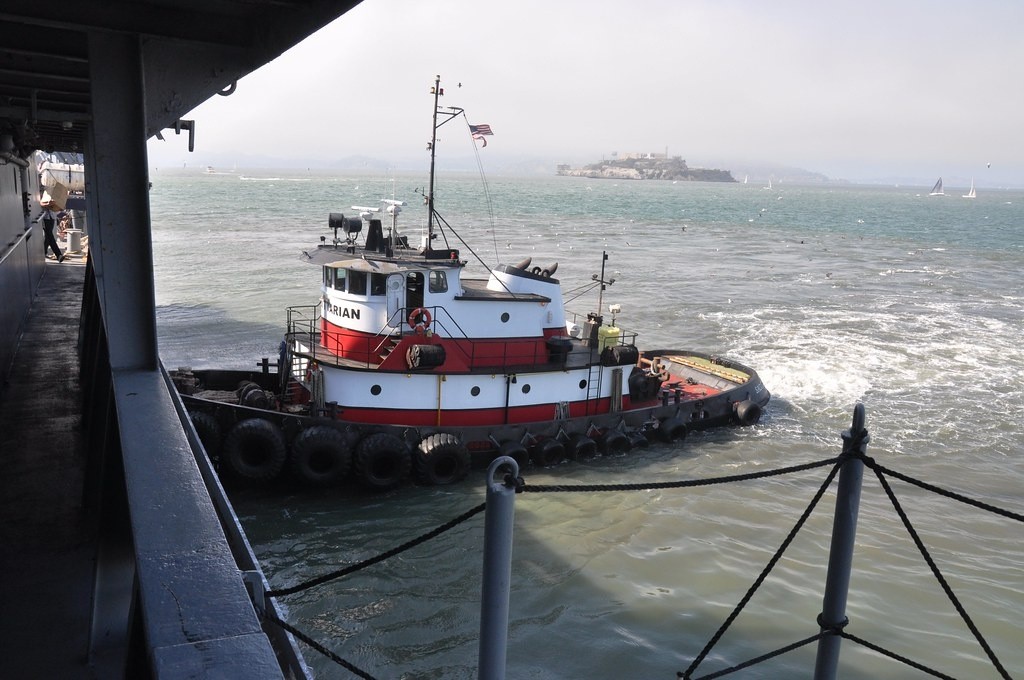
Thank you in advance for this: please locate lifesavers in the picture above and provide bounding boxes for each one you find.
[566,434,597,462]
[290,424,353,491]
[736,400,762,426]
[223,418,287,482]
[659,417,687,444]
[414,431,473,486]
[496,440,530,473]
[534,438,566,467]
[186,409,223,467]
[600,429,633,456]
[352,433,414,491]
[409,307,431,330]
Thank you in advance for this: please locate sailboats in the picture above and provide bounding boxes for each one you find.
[929,177,944,195]
[962,178,976,198]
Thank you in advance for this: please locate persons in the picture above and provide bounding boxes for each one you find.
[39,190,67,263]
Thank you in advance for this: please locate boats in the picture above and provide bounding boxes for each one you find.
[162,73,771,492]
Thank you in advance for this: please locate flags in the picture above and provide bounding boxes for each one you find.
[469,124,494,148]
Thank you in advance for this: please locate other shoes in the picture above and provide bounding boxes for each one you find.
[58,254,64,262]
